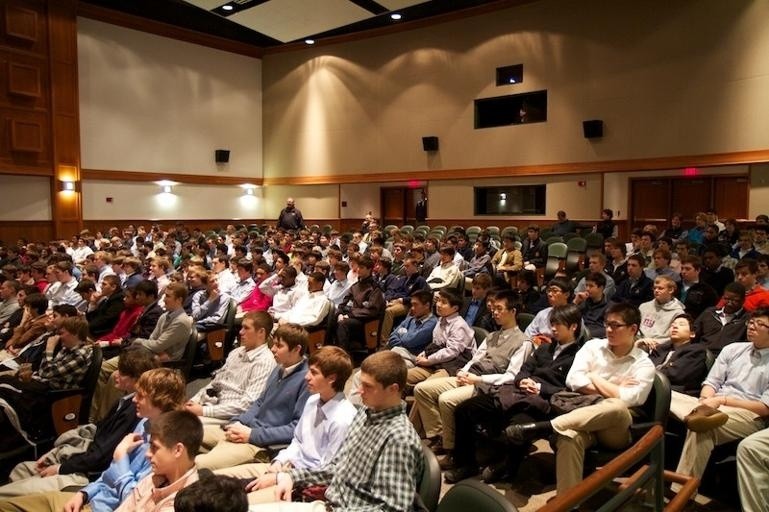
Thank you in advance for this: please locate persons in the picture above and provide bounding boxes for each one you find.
[0,199,769,512]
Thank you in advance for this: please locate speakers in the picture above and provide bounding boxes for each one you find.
[582,120,603,138]
[215,150,230,163]
[423,137,438,150]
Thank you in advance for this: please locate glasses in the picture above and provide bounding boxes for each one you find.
[604,319,628,329]
[745,318,769,330]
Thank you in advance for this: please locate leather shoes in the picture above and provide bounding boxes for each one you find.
[505,420,537,446]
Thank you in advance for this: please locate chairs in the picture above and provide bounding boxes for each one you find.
[0,224,768,511]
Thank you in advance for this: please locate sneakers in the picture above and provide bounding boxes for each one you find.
[431,437,517,483]
[686,404,729,432]
